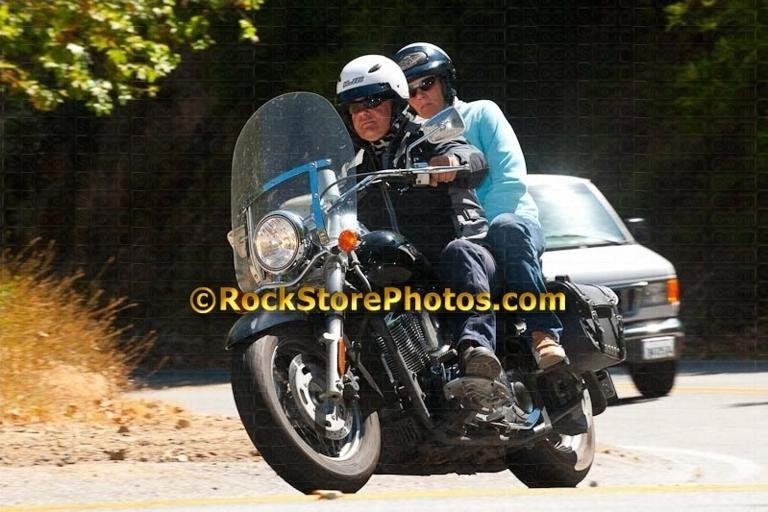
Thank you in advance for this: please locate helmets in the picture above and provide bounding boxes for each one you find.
[336,41,457,101]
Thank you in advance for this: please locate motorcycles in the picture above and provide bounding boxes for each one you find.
[224,92,626,495]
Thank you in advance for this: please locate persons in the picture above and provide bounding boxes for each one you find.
[336,41,566,376]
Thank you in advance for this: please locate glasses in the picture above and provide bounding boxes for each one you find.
[342,96,384,113]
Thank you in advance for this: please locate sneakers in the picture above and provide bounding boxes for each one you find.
[530,331,566,370]
[460,346,502,379]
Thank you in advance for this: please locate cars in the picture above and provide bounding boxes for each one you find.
[527,174,685,398]
